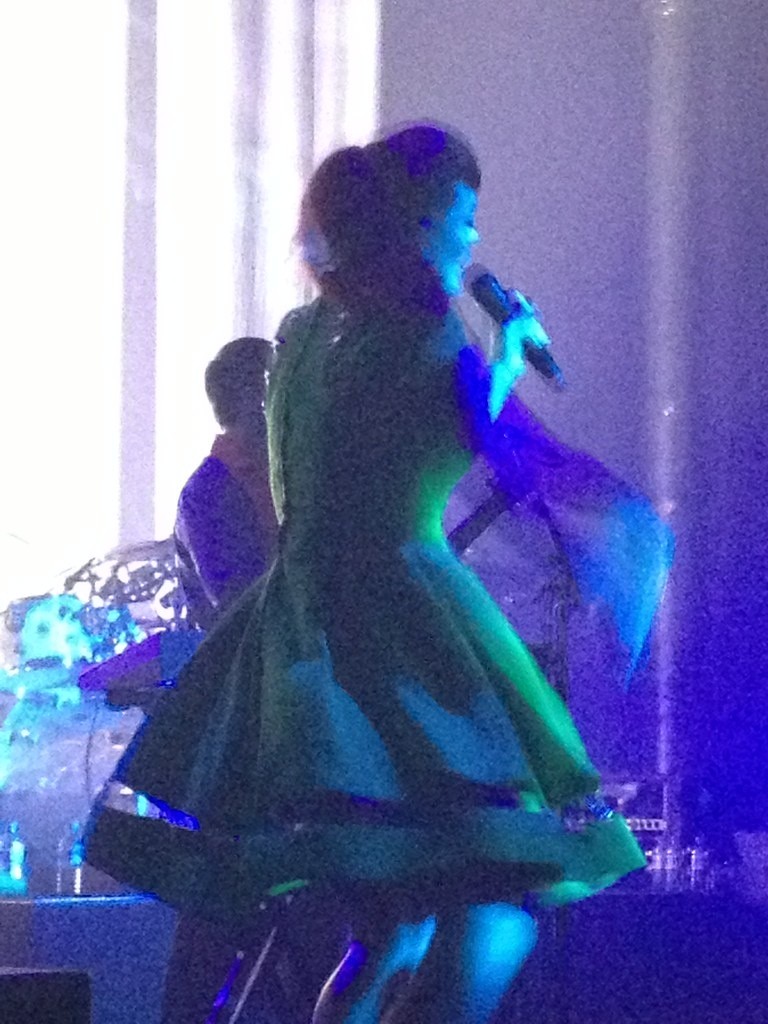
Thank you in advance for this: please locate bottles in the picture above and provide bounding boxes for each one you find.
[56,825,82,899]
[0,821,30,898]
[654,834,707,893]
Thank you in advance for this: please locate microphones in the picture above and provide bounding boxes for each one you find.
[471,275,563,381]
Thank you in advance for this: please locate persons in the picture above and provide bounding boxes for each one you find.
[156,332,276,1024]
[79,124,646,1024]
